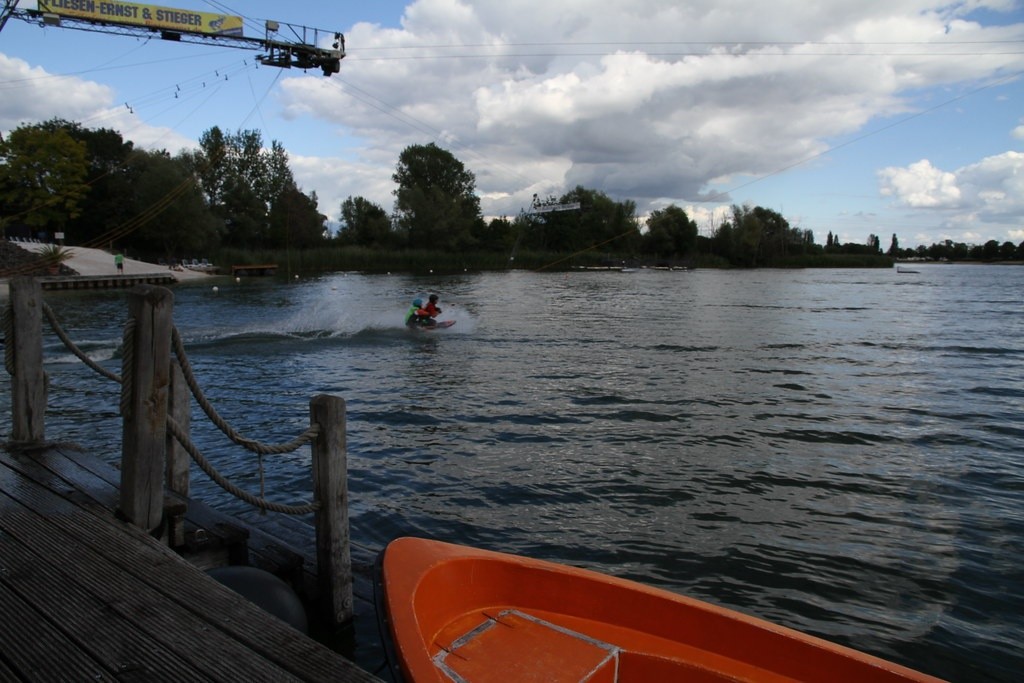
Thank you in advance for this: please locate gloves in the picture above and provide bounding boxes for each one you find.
[436,308,441,313]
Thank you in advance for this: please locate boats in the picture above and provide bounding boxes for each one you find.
[897,265,921,273]
[383,537,950,683]
[416,318,456,331]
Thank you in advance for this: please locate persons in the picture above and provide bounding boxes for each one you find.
[114,251,126,275]
[405,299,429,330]
[420,294,441,326]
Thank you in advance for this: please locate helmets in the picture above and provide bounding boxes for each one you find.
[412,299,422,307]
[429,294,438,303]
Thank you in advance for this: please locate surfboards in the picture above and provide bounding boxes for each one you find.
[423,322,455,330]
